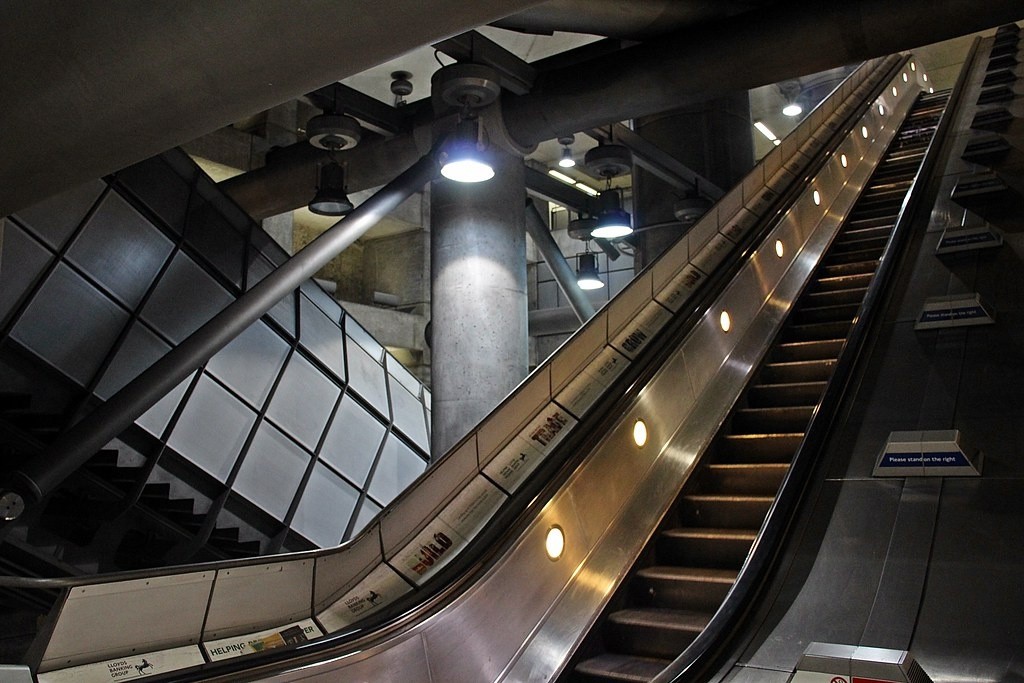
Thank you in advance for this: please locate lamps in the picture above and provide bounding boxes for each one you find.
[558,148,576,167]
[575,252,605,290]
[783,95,803,117]
[439,116,496,184]
[590,191,633,238]
[308,161,355,216]
